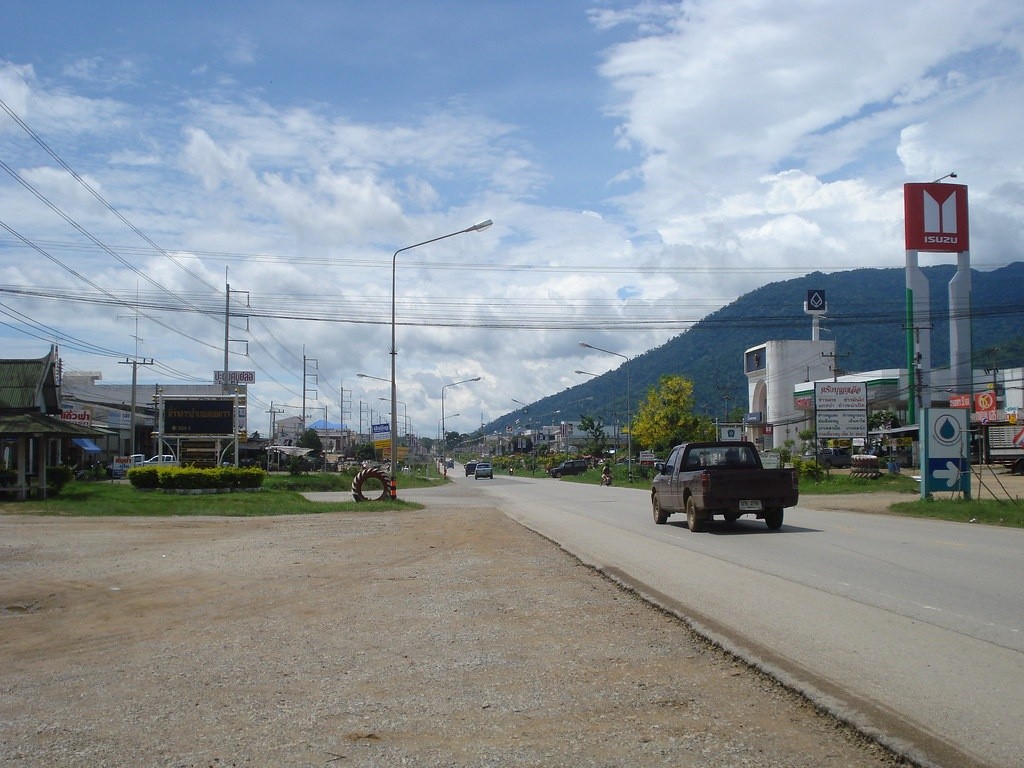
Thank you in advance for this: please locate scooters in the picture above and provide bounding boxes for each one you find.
[601,468,613,487]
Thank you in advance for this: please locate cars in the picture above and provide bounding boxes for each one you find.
[144,454,176,462]
[463,462,479,477]
[474,463,494,480]
[438,455,455,469]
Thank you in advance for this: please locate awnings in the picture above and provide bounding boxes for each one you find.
[866,425,919,462]
[71,439,101,452]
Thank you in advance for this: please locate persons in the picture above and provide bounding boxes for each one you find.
[508,465,512,473]
[594,459,598,469]
[600,463,614,486]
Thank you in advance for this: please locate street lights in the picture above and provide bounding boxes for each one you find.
[390,218,495,502]
[537,410,562,461]
[511,398,536,475]
[388,412,412,451]
[378,396,407,447]
[577,342,634,484]
[566,395,593,460]
[440,376,482,480]
[574,369,618,469]
[438,414,461,474]
[481,422,500,473]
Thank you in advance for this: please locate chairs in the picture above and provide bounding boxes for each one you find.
[723,451,739,465]
[687,454,702,467]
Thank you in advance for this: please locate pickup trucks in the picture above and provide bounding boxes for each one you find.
[800,447,851,470]
[650,441,799,533]
[549,460,588,479]
[106,454,181,479]
